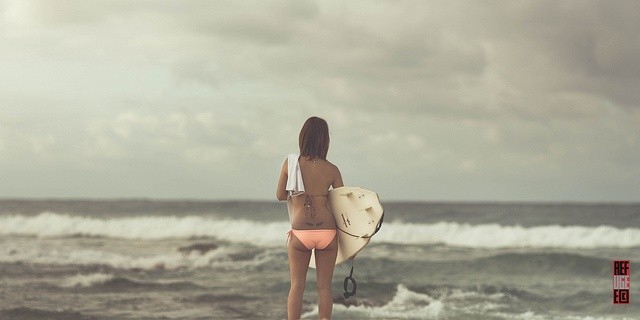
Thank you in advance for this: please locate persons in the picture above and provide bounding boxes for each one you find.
[276,116,343,319]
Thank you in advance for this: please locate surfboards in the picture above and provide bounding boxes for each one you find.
[308,186,383,268]
